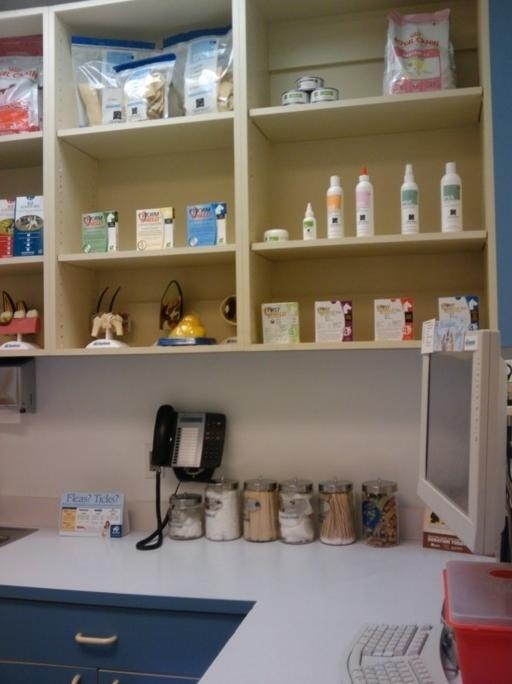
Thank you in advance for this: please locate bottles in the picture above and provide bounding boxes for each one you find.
[302,202,317,242]
[168,475,401,549]
[325,175,344,240]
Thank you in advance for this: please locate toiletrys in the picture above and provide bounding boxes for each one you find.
[295,73,324,90]
[310,86,340,103]
[263,227,290,242]
[302,201,316,239]
[281,89,310,104]
[323,174,347,238]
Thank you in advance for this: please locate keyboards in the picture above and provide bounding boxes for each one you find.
[338,622,448,684]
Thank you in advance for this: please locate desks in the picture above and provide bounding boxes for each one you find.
[197,603,445,681]
[0,527,497,638]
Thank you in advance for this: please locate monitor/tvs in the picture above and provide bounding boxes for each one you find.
[415,329,508,558]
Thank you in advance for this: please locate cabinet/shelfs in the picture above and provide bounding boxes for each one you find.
[0,12,56,356]
[237,0,497,351]
[48,2,247,349]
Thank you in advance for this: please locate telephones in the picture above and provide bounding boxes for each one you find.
[150,404,226,469]
